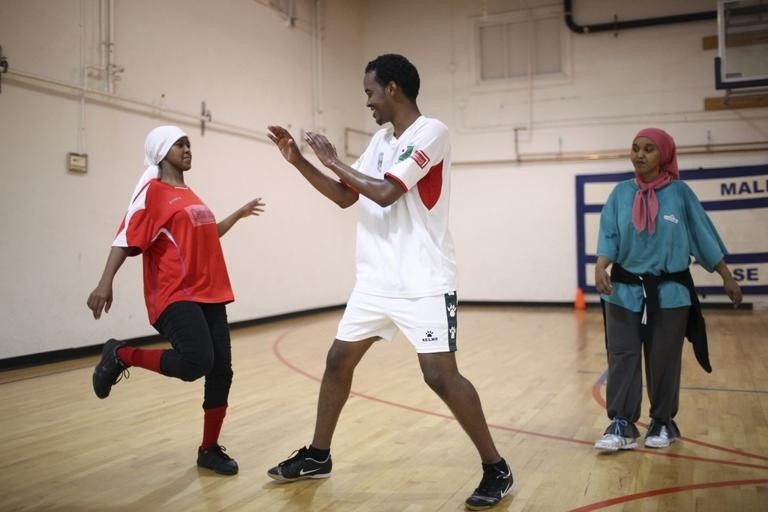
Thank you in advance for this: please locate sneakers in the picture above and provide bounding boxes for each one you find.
[593,433,638,452]
[645,423,678,449]
[464,464,517,511]
[92,338,131,400]
[196,443,239,476]
[266,446,332,483]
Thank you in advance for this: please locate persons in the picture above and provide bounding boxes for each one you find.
[592,125,743,454]
[268,51,515,512]
[84,123,268,477]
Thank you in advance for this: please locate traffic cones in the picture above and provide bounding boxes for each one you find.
[571,287,589,311]
